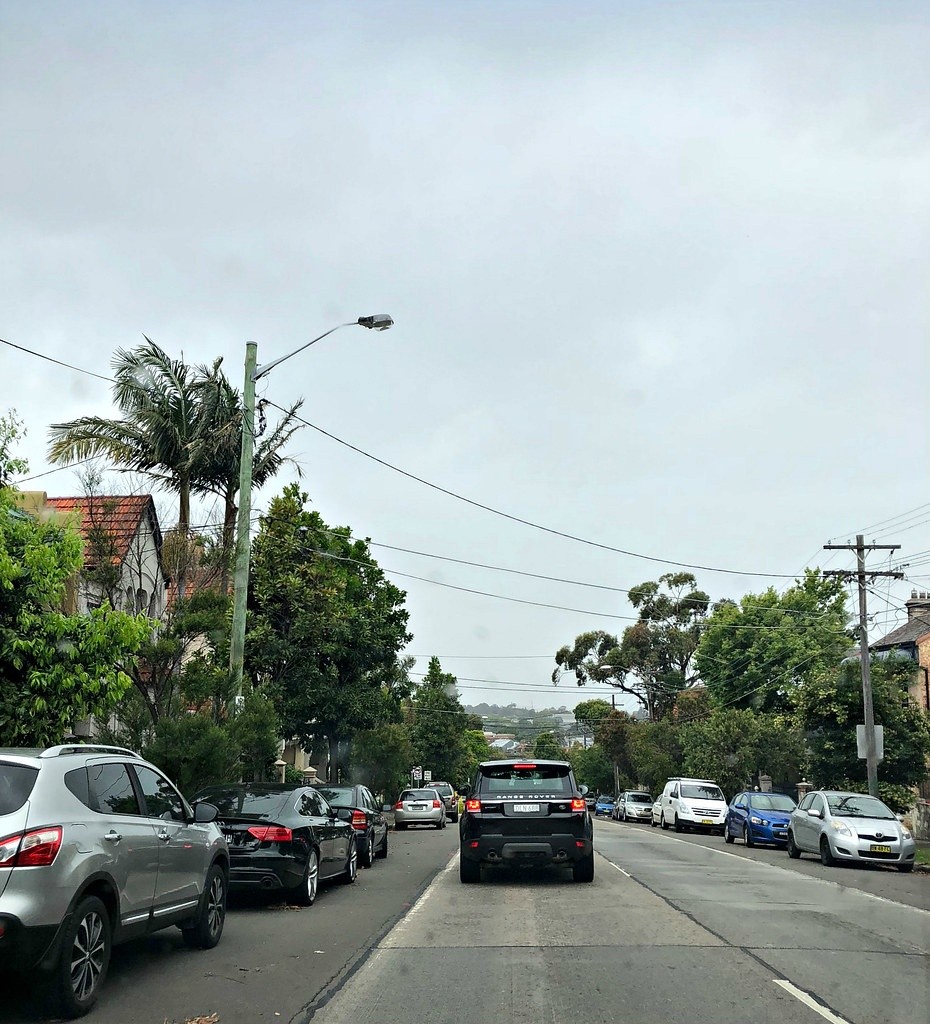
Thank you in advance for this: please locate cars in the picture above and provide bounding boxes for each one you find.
[308,781,388,867]
[187,784,357,906]
[585,789,655,822]
[787,791,917,873]
[724,791,798,850]
[650,794,663,827]
[394,788,447,829]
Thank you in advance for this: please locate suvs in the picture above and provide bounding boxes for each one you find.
[423,780,459,823]
[0,744,230,1017]
[457,759,594,884]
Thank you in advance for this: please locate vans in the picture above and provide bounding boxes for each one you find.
[651,777,729,836]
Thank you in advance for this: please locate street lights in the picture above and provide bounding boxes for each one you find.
[227,313,394,783]
